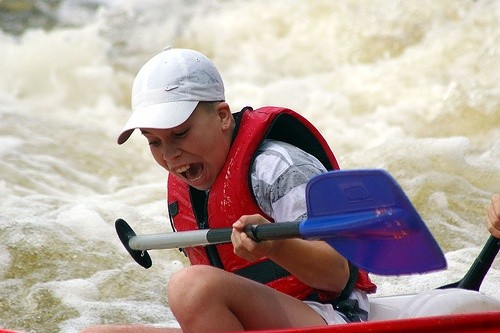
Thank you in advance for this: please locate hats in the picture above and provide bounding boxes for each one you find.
[117,46,225,145]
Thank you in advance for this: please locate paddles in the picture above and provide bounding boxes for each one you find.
[115,168,448,276]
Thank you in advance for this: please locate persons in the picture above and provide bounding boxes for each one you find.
[78,47,377,333]
